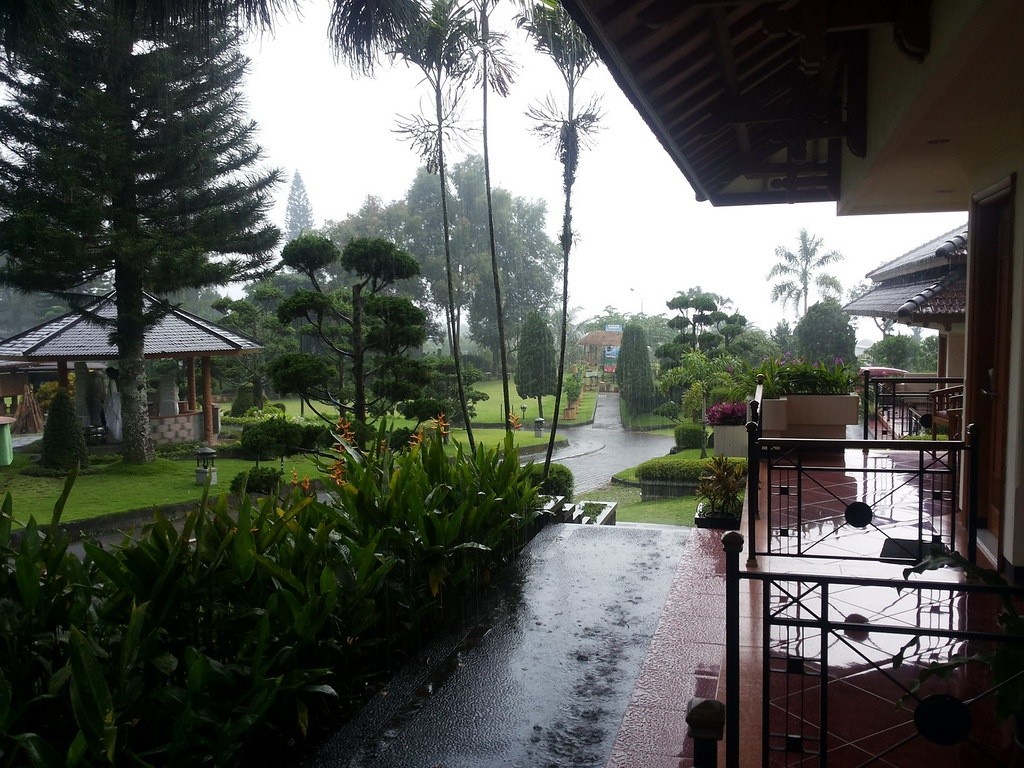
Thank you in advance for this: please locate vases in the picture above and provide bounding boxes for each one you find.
[713,426,749,458]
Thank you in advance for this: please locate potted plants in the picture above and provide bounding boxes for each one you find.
[742,361,859,448]
[694,453,747,530]
[562,367,584,420]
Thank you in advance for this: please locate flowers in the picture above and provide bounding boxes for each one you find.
[706,402,746,426]
